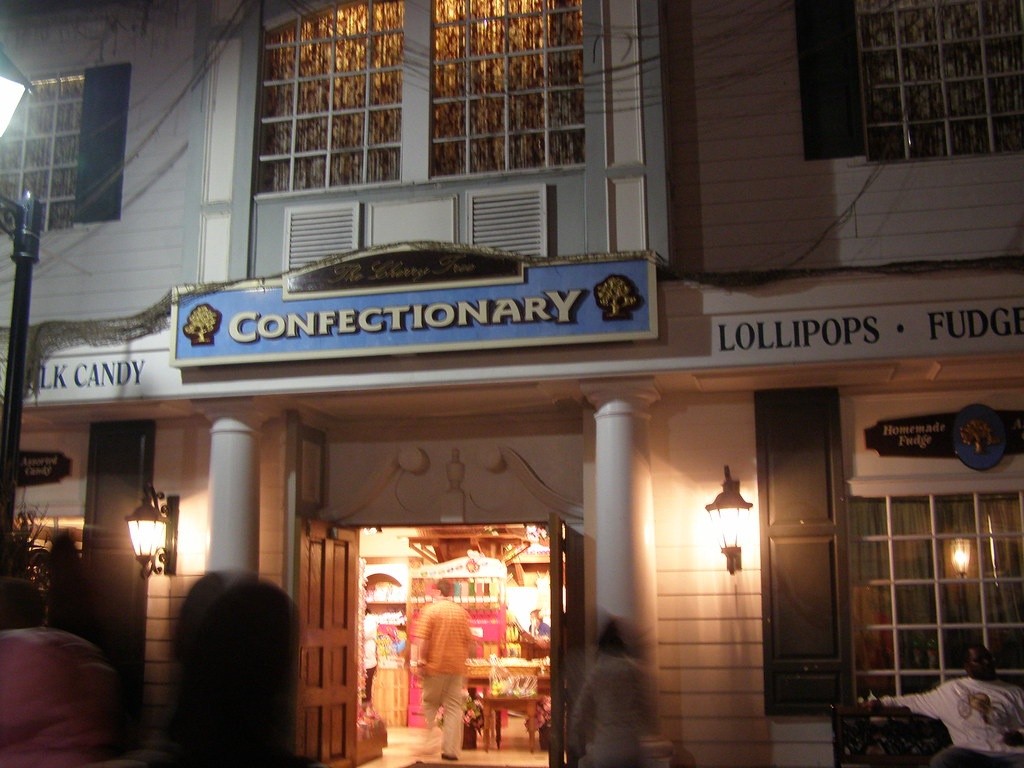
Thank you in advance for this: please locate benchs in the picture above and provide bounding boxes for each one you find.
[829,703,952,768]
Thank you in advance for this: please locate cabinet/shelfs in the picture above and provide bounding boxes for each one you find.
[360,556,423,728]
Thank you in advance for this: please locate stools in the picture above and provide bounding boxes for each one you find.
[481,695,542,754]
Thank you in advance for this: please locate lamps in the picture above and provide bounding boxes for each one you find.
[951,537,973,579]
[123,481,180,581]
[705,464,753,575]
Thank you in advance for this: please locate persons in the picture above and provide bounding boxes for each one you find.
[861,643,1024,768]
[408,579,473,760]
[363,601,379,714]
[135,571,326,768]
[0,578,125,768]
[529,609,551,637]
[567,616,649,768]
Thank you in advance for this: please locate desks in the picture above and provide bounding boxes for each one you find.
[462,676,551,752]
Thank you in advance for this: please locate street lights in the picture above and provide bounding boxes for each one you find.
[0,53,43,613]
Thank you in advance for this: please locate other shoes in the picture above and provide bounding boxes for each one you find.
[441,752,460,761]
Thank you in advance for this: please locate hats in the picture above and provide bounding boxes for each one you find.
[431,578,452,596]
